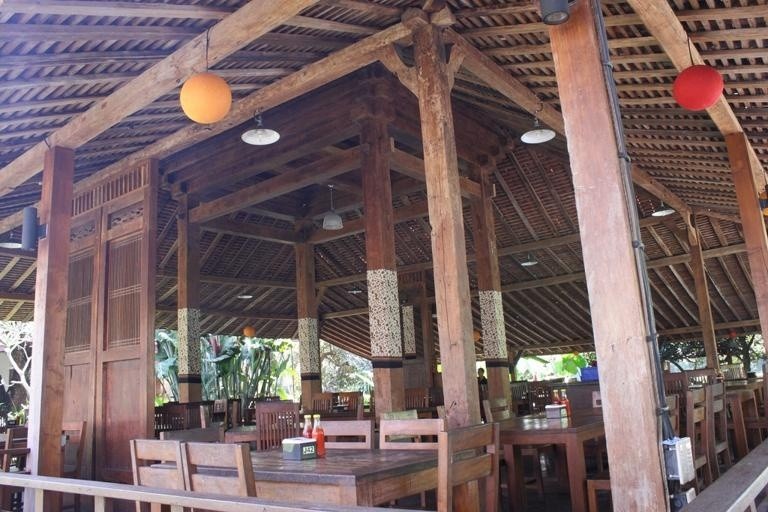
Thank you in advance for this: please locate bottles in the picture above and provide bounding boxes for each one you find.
[311,414,325,457]
[552,389,560,405]
[560,388,570,417]
[303,414,312,438]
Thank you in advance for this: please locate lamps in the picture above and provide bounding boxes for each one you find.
[323,184,344,230]
[243,326,255,337]
[22,207,37,251]
[180,29,232,124]
[237,287,253,299]
[348,283,362,294]
[521,253,538,266]
[240,107,281,145]
[540,0,569,25]
[672,30,724,111]
[521,116,556,144]
[651,200,675,216]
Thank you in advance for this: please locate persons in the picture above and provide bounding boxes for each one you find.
[477,368,487,396]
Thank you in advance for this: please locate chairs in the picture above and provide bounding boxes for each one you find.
[0,421,87,512]
